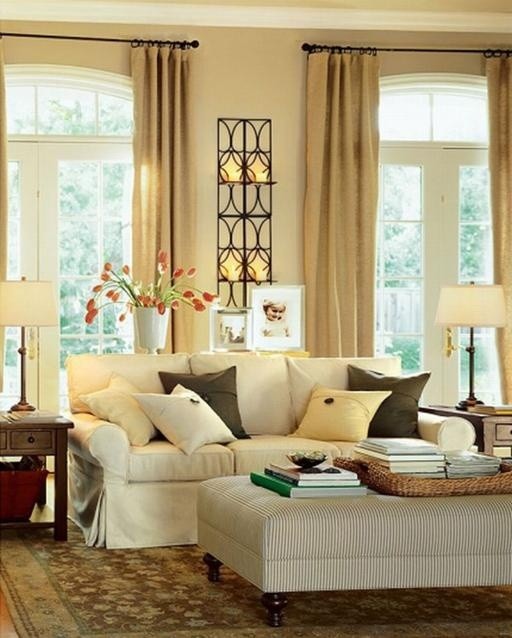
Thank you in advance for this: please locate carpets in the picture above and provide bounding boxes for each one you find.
[0,517,512,638]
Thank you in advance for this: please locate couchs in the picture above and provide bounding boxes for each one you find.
[64,352,477,550]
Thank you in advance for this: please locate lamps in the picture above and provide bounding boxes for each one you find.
[436,281,507,411]
[0,277,58,413]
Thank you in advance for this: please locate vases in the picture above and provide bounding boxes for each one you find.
[135,307,169,354]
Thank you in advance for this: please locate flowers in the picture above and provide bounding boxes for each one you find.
[85,250,214,324]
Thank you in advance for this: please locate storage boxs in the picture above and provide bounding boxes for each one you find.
[1,462,48,522]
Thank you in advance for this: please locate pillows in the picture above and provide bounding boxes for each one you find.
[79,371,161,447]
[158,366,252,439]
[346,364,431,439]
[287,384,392,442]
[131,384,237,456]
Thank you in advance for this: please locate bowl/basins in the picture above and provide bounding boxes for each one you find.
[286,450,329,467]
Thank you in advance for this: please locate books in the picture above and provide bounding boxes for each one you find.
[250,438,501,499]
[468,404,512,416]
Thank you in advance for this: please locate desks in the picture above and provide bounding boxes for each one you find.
[0,415,74,541]
[418,406,512,459]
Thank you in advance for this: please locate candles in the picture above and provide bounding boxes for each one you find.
[229,173,240,181]
[229,271,239,281]
[256,271,266,281]
[256,173,266,182]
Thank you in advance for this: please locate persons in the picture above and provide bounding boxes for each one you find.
[261,297,289,337]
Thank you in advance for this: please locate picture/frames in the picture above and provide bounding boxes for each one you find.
[209,308,253,352]
[248,285,306,353]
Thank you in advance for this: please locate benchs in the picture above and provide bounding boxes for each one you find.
[197,475,512,627]
